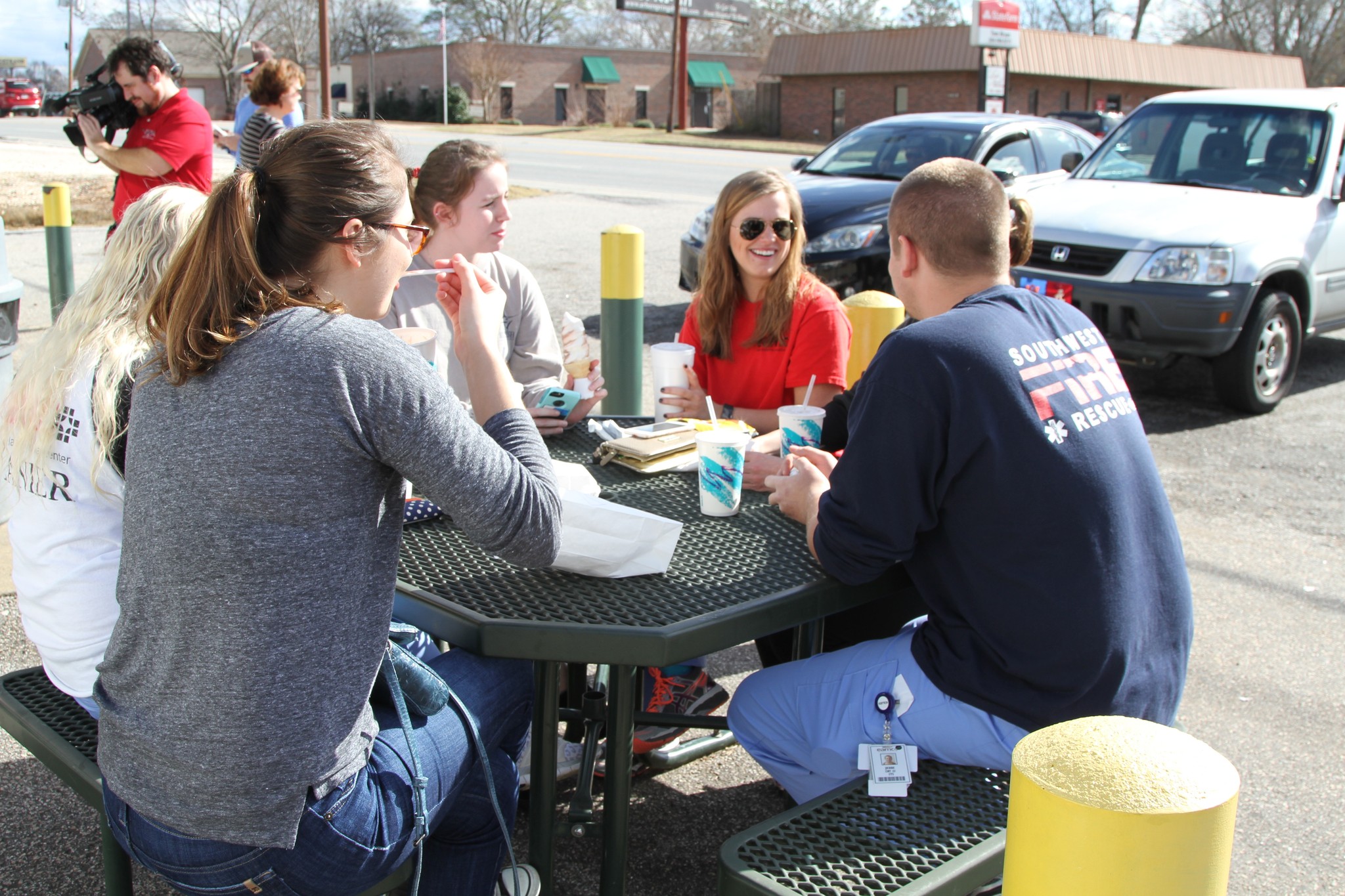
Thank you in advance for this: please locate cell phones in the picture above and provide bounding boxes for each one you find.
[625,420,695,439]
[536,388,581,421]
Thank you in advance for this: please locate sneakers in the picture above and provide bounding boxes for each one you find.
[594,738,651,781]
[633,667,729,754]
[518,720,583,791]
[495,864,541,896]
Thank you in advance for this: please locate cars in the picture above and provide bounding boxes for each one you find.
[1001,86,1345,411]
[677,112,1144,305]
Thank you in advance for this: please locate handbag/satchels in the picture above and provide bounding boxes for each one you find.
[372,621,522,896]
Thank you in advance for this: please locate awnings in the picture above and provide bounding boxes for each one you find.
[686,60,735,89]
[582,56,620,84]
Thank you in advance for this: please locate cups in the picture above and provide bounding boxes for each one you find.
[650,342,696,423]
[696,430,751,517]
[777,405,826,459]
[405,480,412,499]
[389,327,437,367]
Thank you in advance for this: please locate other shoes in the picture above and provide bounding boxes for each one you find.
[966,873,1002,896]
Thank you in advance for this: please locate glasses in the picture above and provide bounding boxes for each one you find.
[322,221,430,255]
[291,84,303,94]
[731,218,798,241]
[243,60,264,75]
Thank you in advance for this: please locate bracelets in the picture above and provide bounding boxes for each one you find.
[721,403,734,419]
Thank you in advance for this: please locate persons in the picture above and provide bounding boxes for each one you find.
[371,141,610,437]
[240,56,306,174]
[209,39,305,169]
[589,170,855,785]
[728,155,1196,896]
[91,121,563,896]
[0,182,604,791]
[883,755,896,765]
[73,36,213,258]
[742,192,1032,666]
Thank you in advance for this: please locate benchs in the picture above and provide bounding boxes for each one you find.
[0,668,133,896]
[713,759,1011,896]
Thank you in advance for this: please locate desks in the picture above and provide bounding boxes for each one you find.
[402,411,892,894]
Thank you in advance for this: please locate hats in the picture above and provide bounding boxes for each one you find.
[227,40,272,73]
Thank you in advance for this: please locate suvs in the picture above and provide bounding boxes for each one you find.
[0,77,41,118]
